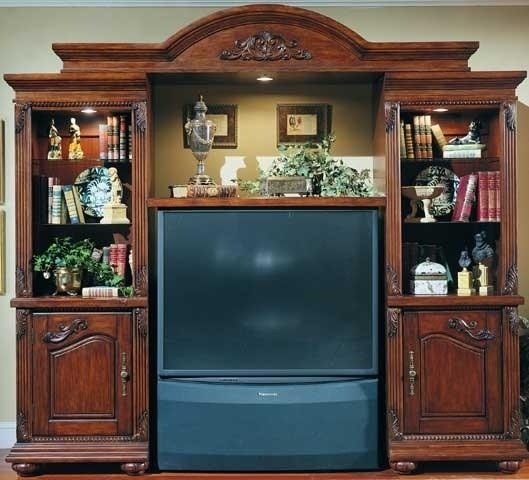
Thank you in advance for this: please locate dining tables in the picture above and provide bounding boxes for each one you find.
[232,133,374,198]
[32,236,132,296]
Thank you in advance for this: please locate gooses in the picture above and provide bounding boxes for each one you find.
[181,94,217,186]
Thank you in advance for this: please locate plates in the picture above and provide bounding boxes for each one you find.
[3,4,529,477]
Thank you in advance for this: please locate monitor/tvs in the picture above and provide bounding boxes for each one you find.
[167,184,239,199]
[399,114,502,223]
[46,114,132,298]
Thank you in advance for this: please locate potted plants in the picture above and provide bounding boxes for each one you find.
[276,102,329,148]
[183,103,238,149]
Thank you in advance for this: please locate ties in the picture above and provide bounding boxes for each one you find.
[158,211,378,383]
